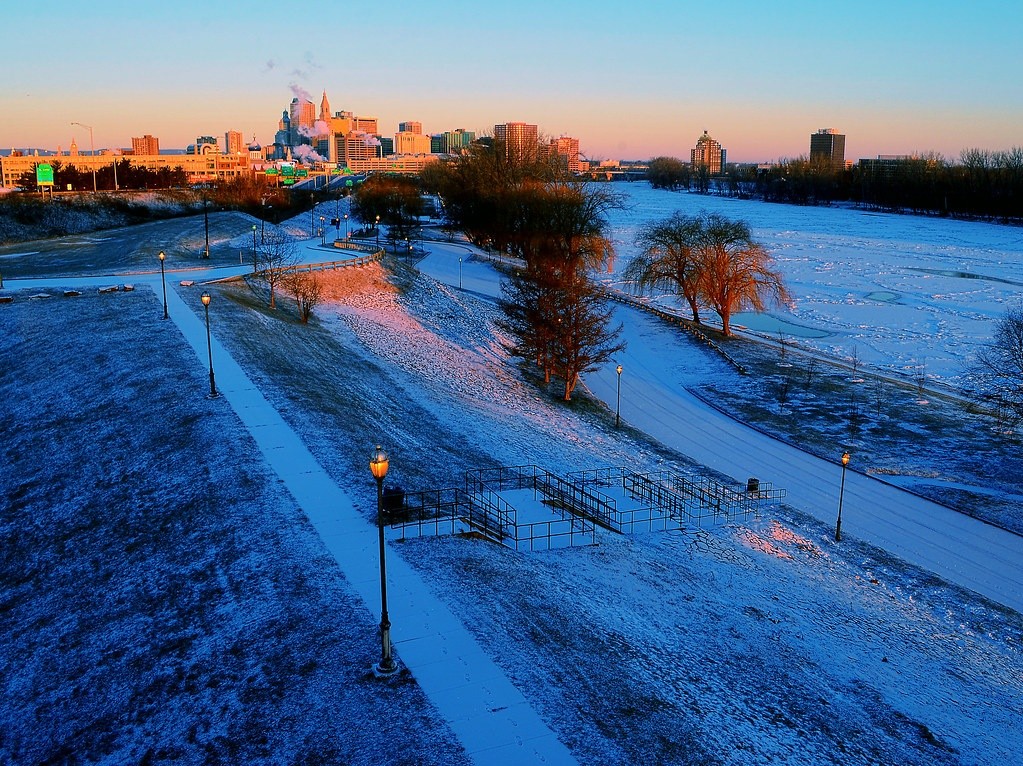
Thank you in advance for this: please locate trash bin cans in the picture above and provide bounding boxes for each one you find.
[384,485,406,511]
[331,219,336,225]
[747,478,759,491]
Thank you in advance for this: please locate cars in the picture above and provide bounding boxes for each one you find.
[186,183,219,192]
[11,184,61,193]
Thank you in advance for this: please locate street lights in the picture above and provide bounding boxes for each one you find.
[158,251,169,319]
[409,245,412,266]
[319,216,326,246]
[375,215,380,252]
[615,364,623,429]
[201,290,220,398]
[251,224,257,272]
[835,451,850,541]
[71,122,96,195]
[404,238,408,263]
[261,205,273,245]
[368,443,403,681]
[311,201,320,235]
[344,214,348,249]
[459,257,462,289]
[336,194,343,240]
